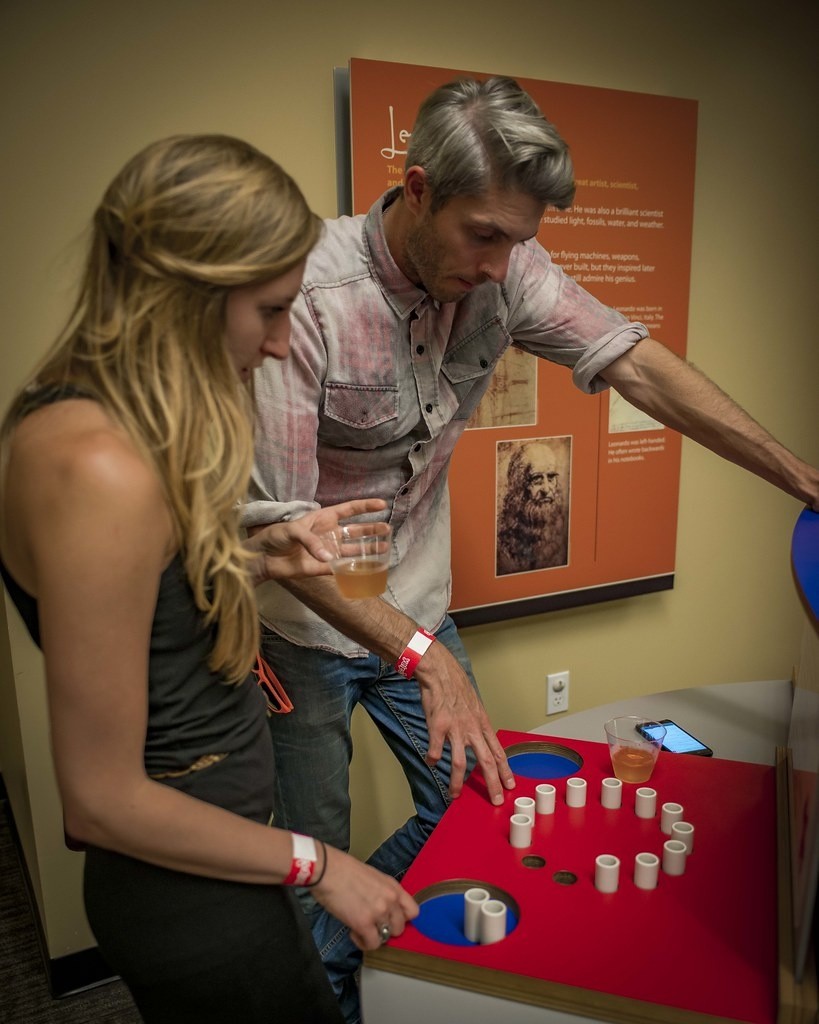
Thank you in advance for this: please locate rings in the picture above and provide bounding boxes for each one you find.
[379,923,392,941]
[342,525,353,545]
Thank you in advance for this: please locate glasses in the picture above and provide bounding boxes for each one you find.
[250,650,294,714]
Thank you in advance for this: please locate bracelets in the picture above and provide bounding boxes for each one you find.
[307,837,328,888]
[283,829,318,888]
[393,623,437,679]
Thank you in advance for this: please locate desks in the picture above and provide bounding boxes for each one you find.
[352,679,819,1023]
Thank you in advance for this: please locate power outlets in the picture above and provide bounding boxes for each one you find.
[545,670,569,716]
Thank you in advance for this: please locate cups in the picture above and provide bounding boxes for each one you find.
[601,712,668,785]
[321,519,393,603]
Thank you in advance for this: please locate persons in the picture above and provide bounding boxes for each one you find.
[237,73,819,1024]
[1,130,397,1024]
[495,441,568,577]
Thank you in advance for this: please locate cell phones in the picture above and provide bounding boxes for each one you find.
[636,719,713,757]
